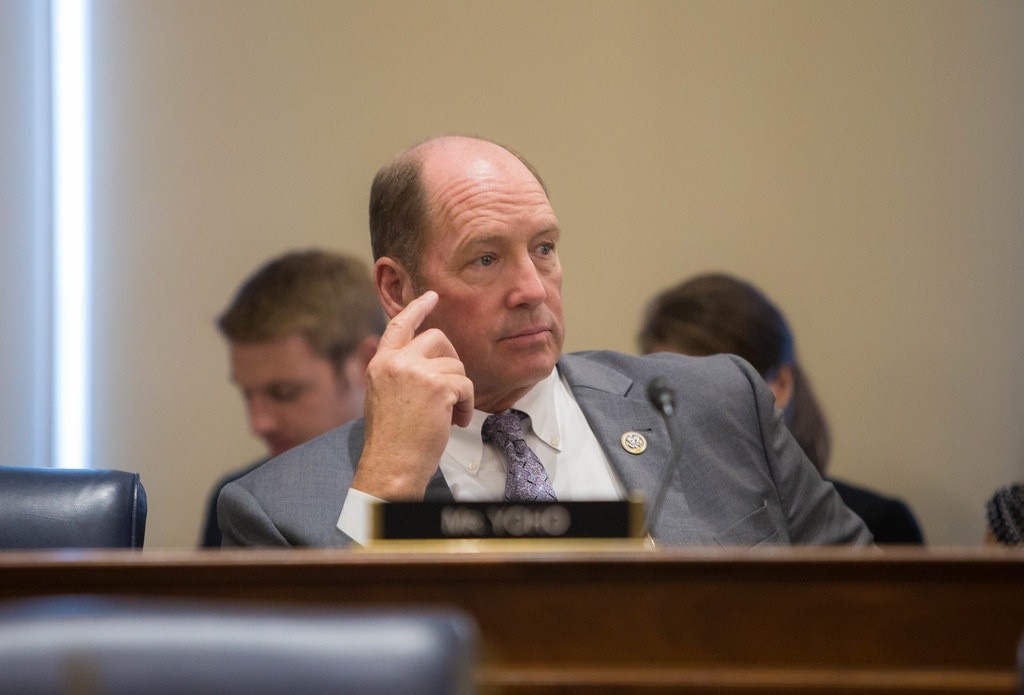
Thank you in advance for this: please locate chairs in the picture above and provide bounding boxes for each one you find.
[0,464,147,549]
[0,596,474,695]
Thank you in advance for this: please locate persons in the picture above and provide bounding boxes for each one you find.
[218,130,878,553]
[636,273,924,548]
[200,248,397,552]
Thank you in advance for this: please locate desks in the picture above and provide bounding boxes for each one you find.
[0,545,1024,695]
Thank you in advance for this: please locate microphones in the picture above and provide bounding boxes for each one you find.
[638,376,683,542]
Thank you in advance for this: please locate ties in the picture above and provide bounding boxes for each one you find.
[485,410,556,502]
